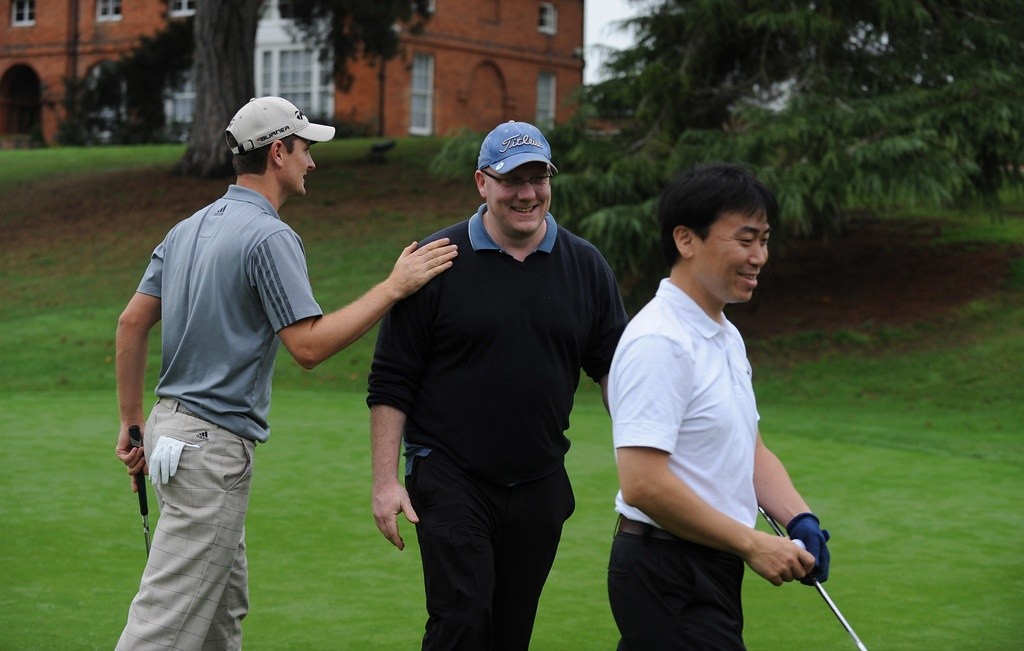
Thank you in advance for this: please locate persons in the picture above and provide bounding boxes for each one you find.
[605,163,830,651]
[115,97,458,651]
[365,117,635,651]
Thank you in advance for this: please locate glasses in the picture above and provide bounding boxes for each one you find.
[481,169,553,188]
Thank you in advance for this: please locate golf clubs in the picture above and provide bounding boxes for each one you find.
[128,422,151,559]
[760,504,868,651]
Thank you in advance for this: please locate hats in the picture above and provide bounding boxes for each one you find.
[225,96,335,154]
[478,120,559,174]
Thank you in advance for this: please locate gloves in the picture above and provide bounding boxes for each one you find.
[147,437,186,486]
[786,513,831,586]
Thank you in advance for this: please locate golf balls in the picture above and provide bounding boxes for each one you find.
[792,539,807,551]
